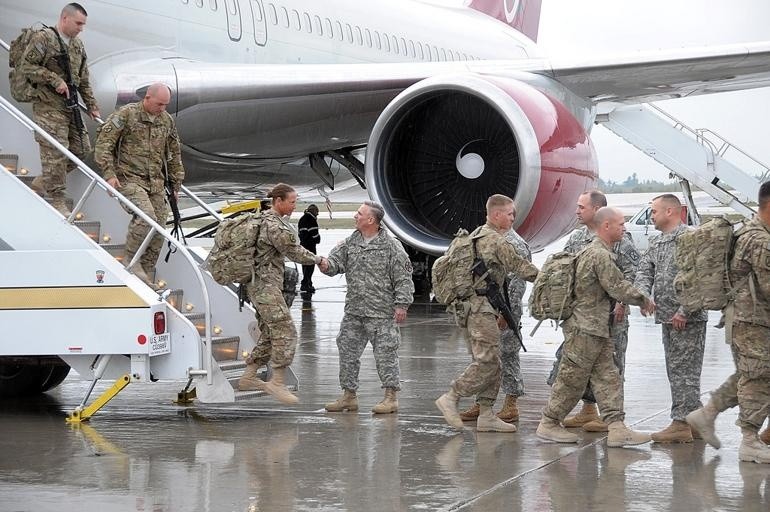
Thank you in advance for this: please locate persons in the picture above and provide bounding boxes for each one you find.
[238,183,327,405]
[561,189,641,432]
[461,205,533,422]
[297,204,321,293]
[535,205,657,447]
[436,194,541,432]
[686,181,770,464]
[634,193,709,444]
[20,3,100,220]
[94,84,185,283]
[317,199,415,415]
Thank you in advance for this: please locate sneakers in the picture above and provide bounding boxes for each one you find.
[125,258,148,283]
[146,282,161,290]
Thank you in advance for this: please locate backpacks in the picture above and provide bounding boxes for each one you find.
[528,244,606,320]
[9,26,60,102]
[209,212,279,286]
[674,216,764,311]
[432,227,502,305]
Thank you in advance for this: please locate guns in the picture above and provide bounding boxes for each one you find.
[236,282,250,312]
[470,258,528,352]
[51,53,85,154]
[163,178,187,246]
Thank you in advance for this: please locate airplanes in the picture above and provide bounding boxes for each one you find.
[0,1,769,398]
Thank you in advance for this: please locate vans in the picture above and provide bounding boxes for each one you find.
[618,204,687,254]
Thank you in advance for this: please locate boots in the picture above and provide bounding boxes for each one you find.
[49,196,70,217]
[265,367,300,404]
[652,397,722,449]
[740,417,770,464]
[238,364,266,390]
[435,388,519,432]
[372,388,399,413]
[33,175,46,192]
[537,403,652,447]
[325,389,358,412]
